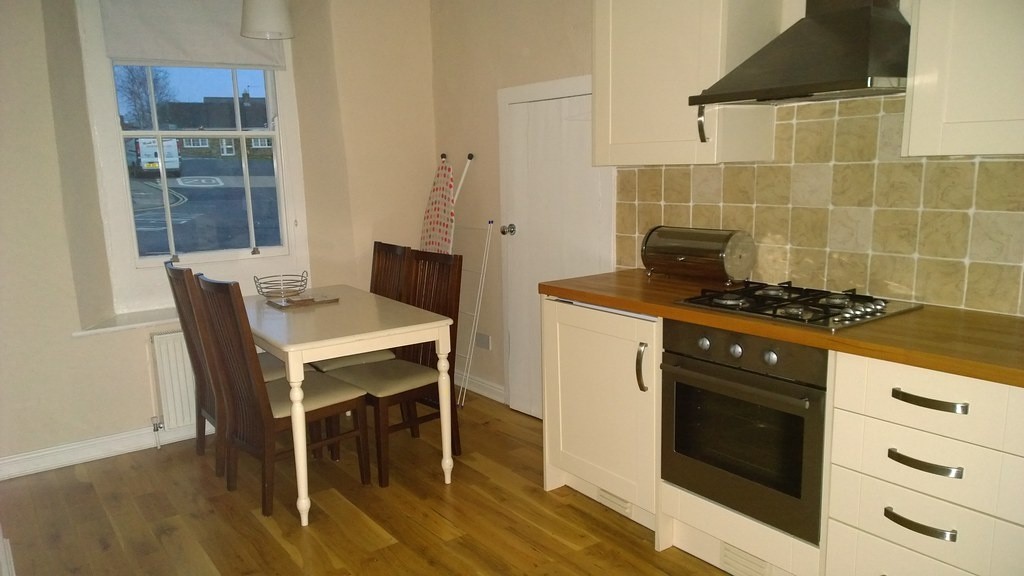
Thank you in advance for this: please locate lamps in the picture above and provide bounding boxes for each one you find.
[241,0,294,43]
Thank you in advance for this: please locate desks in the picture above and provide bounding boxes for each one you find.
[242,284,454,526]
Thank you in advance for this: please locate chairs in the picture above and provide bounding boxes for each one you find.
[163,260,322,458]
[194,273,373,517]
[325,251,463,488]
[330,240,410,463]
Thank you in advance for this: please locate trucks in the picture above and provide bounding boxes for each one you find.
[125,137,184,178]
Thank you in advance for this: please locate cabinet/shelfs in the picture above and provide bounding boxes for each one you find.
[593,0,782,166]
[901,0,1024,158]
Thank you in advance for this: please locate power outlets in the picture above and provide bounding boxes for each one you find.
[475,332,492,349]
[540,301,1024,576]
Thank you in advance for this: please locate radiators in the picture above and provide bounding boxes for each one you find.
[146,331,209,431]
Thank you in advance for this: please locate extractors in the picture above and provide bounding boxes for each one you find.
[688,0,911,107]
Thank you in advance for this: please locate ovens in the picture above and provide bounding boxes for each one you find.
[662,318,829,548]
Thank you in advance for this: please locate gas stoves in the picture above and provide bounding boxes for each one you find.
[675,280,923,331]
